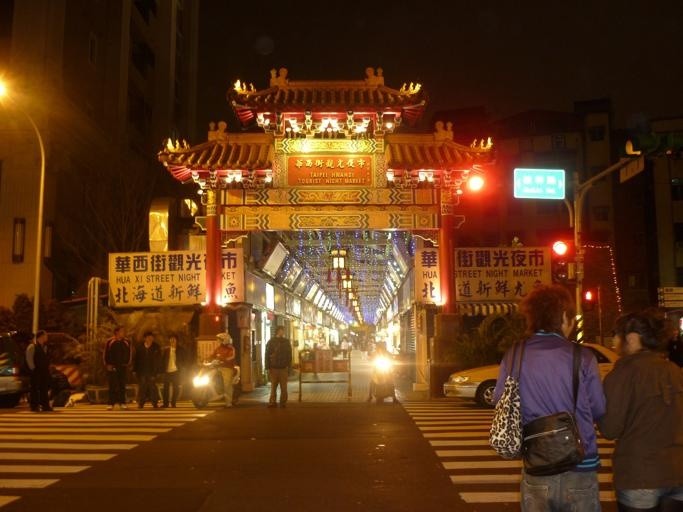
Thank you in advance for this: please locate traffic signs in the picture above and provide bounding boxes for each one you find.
[511,167,568,203]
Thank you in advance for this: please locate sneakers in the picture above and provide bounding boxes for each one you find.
[170,404,176,408]
[225,404,234,408]
[105,405,113,410]
[279,404,287,409]
[267,402,278,409]
[153,404,159,408]
[118,404,127,409]
[364,395,372,403]
[138,406,144,408]
[159,404,169,408]
[392,398,400,404]
[29,404,54,413]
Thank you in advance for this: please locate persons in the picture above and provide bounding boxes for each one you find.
[26,330,54,413]
[133,330,162,409]
[160,333,187,409]
[101,324,132,411]
[262,325,292,408]
[196,332,237,409]
[594,309,682,512]
[339,334,399,405]
[489,284,607,512]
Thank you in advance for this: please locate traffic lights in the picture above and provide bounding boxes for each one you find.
[551,240,575,261]
[583,288,597,311]
[617,131,655,160]
[464,175,490,194]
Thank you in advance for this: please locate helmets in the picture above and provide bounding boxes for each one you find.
[214,333,233,345]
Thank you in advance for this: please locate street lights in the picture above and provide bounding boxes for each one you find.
[0,81,49,348]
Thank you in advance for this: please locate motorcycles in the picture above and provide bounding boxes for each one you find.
[368,360,395,404]
[191,359,240,409]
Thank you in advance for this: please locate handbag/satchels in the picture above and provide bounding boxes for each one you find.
[519,410,586,479]
[487,337,526,459]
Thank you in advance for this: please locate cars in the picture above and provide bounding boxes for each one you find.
[0,331,83,406]
[441,341,622,408]
